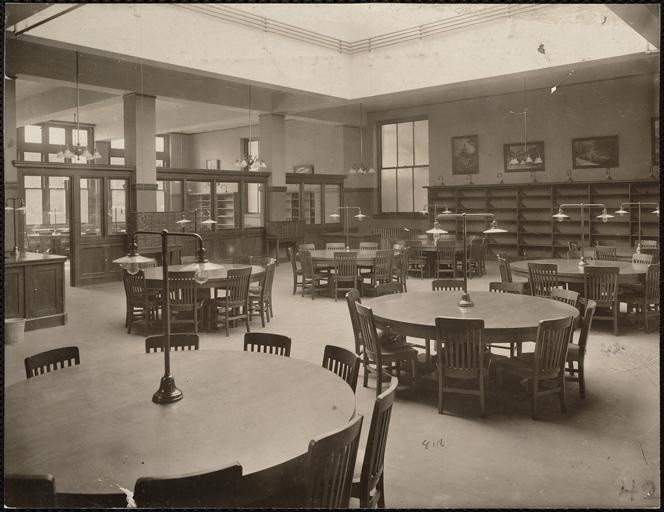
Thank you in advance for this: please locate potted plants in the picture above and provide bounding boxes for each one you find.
[572,135,619,171]
[649,117,660,167]
[450,135,479,175]
[502,140,546,173]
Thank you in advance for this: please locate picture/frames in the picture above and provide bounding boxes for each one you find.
[649,117,660,167]
[450,135,479,175]
[502,140,546,173]
[572,135,619,171]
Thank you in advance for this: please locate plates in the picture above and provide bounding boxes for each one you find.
[421,178,659,262]
[186,192,239,230]
[285,191,316,226]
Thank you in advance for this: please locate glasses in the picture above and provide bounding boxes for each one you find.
[111,230,226,405]
[424,212,508,307]
[54,50,102,161]
[551,201,660,268]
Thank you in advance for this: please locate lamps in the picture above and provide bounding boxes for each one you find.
[111,230,226,405]
[54,50,102,161]
[551,201,660,268]
[424,212,508,307]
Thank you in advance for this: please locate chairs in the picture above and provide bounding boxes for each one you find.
[498,241,658,336]
[4,332,398,512]
[289,227,487,302]
[344,279,596,420]
[121,230,274,336]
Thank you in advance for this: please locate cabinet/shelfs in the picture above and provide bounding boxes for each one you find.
[0,252,67,333]
[285,191,316,226]
[186,192,239,230]
[421,178,659,262]
